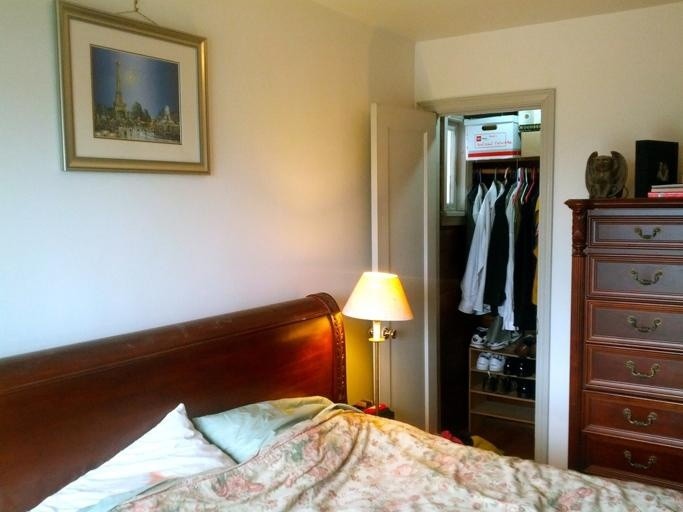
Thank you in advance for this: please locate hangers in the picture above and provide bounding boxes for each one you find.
[466,166,536,205]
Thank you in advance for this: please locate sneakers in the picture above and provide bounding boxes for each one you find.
[482,374,535,401]
[476,352,536,377]
[469,326,536,359]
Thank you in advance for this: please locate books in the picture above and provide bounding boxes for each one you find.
[648,184,683,198]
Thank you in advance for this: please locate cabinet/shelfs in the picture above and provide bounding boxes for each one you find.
[562,198,682,493]
[468,347,535,461]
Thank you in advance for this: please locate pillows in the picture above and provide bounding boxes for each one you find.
[191,396,334,467]
[26,401,238,512]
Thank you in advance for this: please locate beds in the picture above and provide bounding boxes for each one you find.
[0,292,681,512]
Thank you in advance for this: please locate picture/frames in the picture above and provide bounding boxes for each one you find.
[56,1,210,174]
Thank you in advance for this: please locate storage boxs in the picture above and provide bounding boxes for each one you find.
[463,109,541,160]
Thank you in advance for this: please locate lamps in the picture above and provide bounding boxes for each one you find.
[340,271,413,417]
